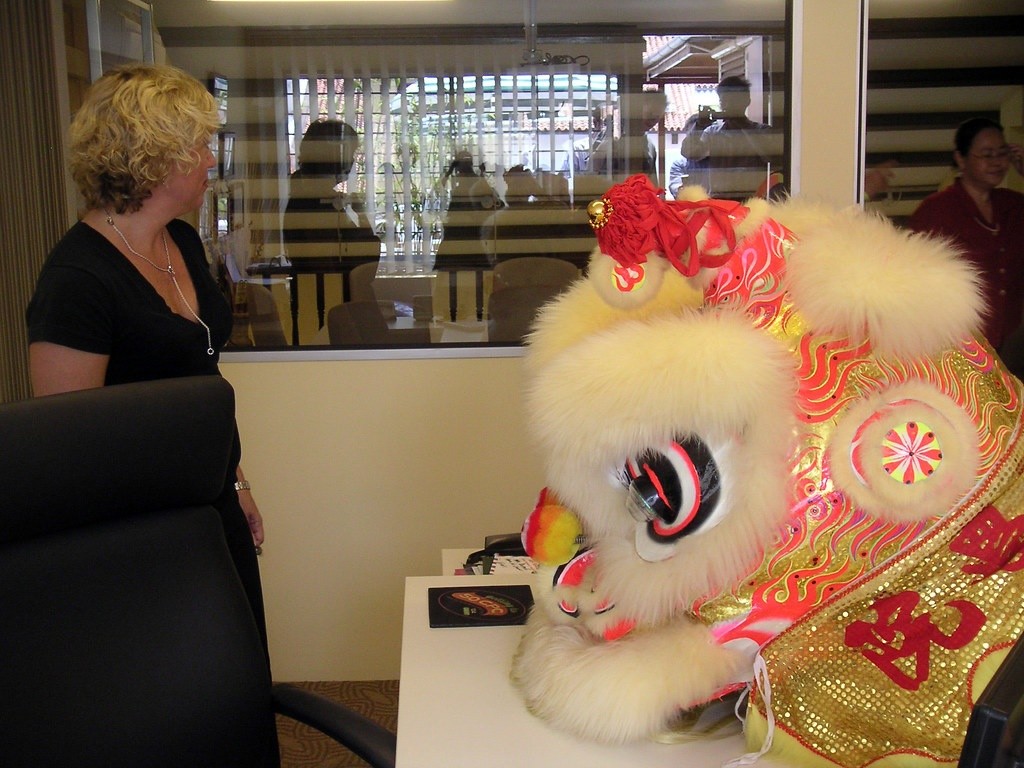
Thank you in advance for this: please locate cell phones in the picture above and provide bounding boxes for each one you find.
[1007,144,1021,162]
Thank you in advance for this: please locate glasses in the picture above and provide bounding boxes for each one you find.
[967,144,1011,162]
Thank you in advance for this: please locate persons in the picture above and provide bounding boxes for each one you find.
[910,114,1024,381]
[434,76,784,322]
[27,69,266,550]
[282,119,381,346]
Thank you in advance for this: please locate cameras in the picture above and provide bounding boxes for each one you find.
[713,112,725,119]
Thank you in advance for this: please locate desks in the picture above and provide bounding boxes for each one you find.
[316,319,490,344]
[396,575,763,768]
[441,548,485,576]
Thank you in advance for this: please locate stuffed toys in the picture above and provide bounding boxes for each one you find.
[511,173,1023,768]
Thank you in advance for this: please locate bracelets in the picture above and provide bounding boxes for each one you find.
[234,480,250,492]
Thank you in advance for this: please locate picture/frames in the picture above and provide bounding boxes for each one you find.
[195,75,244,242]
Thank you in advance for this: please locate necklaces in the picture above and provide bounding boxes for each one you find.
[973,211,1000,234]
[104,206,214,356]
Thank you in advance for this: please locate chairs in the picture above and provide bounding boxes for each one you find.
[328,260,433,345]
[0,376,397,768]
[487,259,579,343]
